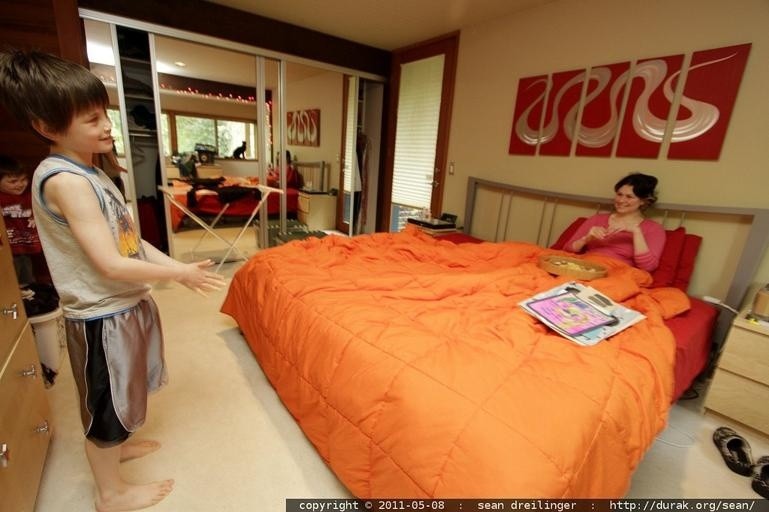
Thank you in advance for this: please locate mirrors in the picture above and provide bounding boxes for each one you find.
[75,5,358,266]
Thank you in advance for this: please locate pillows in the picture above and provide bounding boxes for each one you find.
[675,231,703,292]
[548,217,686,291]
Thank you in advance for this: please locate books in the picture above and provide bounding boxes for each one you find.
[522,279,648,346]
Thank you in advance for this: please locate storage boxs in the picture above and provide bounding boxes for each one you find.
[274,231,325,245]
[254,218,308,248]
[25,308,61,390]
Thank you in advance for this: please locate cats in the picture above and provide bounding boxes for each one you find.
[225,140,246,161]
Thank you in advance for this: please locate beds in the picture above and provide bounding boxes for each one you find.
[223,175,759,508]
[168,156,324,232]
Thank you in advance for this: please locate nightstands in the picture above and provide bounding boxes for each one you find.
[407,216,463,234]
[298,188,336,231]
[700,306,769,439]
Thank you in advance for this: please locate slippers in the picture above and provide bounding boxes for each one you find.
[713,426,752,475]
[752,455,768,498]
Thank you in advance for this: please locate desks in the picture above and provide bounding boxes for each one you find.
[155,181,284,277]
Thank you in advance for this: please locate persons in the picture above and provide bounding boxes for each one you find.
[269,149,298,186]
[0,157,46,298]
[1,48,229,512]
[562,171,667,275]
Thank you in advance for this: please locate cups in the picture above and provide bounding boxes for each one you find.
[302,181,312,191]
[417,206,431,220]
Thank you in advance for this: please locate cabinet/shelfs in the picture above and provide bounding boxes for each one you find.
[0,217,56,511]
